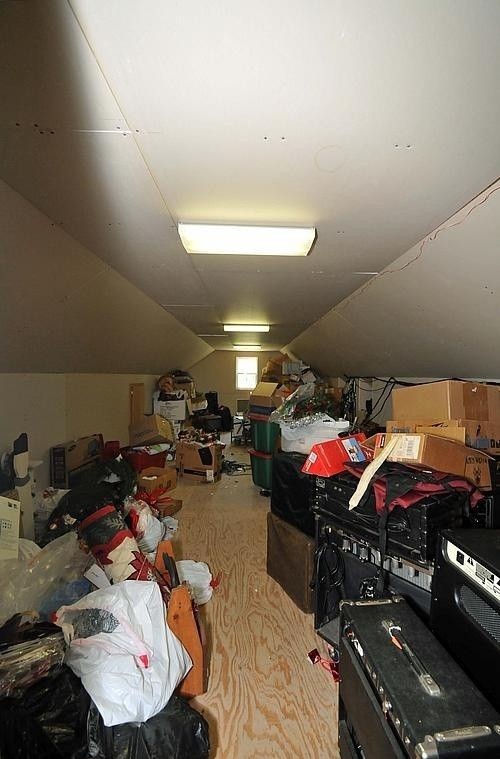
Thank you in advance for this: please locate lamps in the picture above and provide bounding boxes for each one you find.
[177,223,318,257]
[232,345,262,352]
[223,324,270,333]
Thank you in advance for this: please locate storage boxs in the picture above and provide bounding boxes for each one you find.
[128,380,222,498]
[372,380,500,491]
[50,433,104,489]
[247,412,281,454]
[250,353,314,409]
[247,448,273,490]
